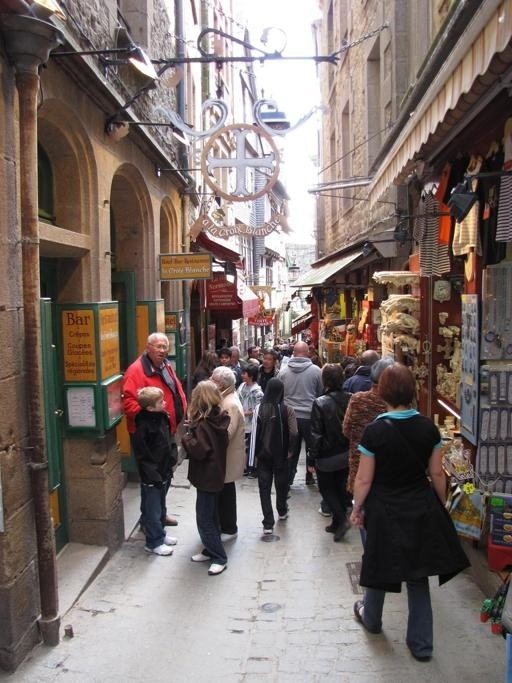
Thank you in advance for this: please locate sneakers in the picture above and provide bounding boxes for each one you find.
[208,562,227,575]
[219,531,238,544]
[286,479,314,497]
[279,513,289,520]
[263,527,274,534]
[318,507,353,542]
[191,552,211,562]
[406,639,433,661]
[354,600,382,633]
[143,544,174,557]
[163,536,177,545]
[164,515,179,526]
[242,469,259,480]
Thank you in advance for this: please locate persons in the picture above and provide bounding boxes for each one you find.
[277,341,395,549]
[351,363,473,662]
[123,333,298,577]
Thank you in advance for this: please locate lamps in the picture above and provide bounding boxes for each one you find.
[49,46,161,84]
[392,208,450,241]
[286,286,368,312]
[105,122,222,207]
[211,256,237,284]
[360,239,395,256]
[446,171,512,224]
